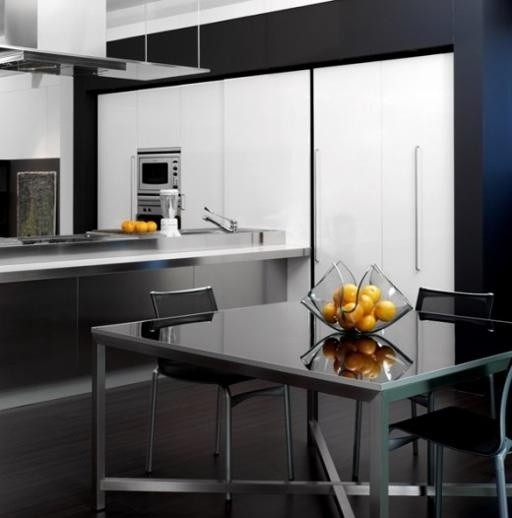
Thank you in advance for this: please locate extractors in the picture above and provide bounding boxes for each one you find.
[0,0,211,82]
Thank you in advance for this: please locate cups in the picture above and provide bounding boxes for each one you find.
[158,188,178,218]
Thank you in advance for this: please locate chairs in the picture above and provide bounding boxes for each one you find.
[350,286,501,486]
[144,284,297,502]
[374,363,512,515]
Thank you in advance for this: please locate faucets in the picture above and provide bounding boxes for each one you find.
[83,230,110,240]
[201,205,237,232]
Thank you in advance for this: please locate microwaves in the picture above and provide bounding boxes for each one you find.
[136,148,181,229]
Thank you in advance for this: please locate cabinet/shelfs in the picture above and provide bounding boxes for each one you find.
[138,84,180,148]
[313,42,454,303]
[92,90,137,233]
[179,81,226,231]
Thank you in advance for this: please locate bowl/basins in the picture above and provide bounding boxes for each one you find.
[297,259,413,334]
[299,330,412,383]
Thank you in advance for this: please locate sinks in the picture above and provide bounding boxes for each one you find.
[179,229,215,236]
[20,234,93,246]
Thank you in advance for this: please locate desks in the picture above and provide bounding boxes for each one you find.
[88,296,510,518]
